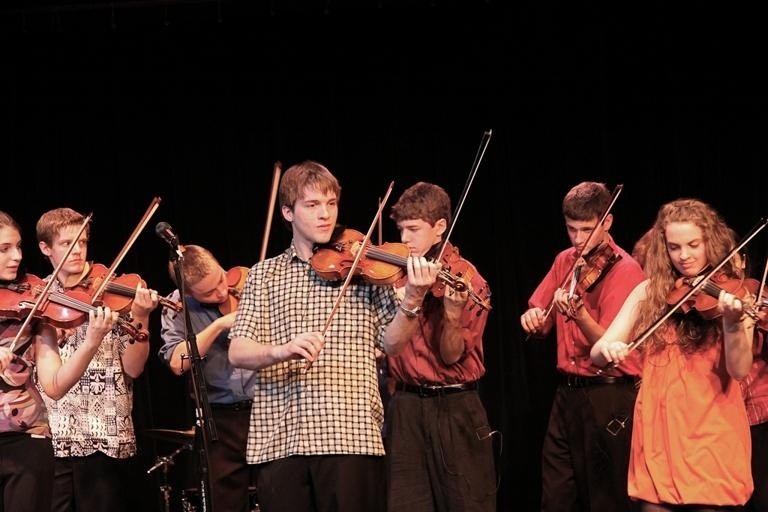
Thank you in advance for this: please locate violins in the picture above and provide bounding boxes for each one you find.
[311,224,469,292]
[220,267,249,315]
[430,240,492,312]
[0,273,150,344]
[65,264,183,314]
[665,275,766,324]
[567,243,614,315]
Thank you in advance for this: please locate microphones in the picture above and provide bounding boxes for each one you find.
[155,220,181,250]
[145,440,195,476]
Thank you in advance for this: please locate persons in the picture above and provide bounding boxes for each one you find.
[228,163,443,512]
[727,238,768,510]
[32,208,158,512]
[590,200,756,512]
[0,211,119,512]
[374,182,495,512]
[157,245,254,511]
[520,182,644,512]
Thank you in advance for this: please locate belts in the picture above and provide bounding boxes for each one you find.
[559,373,632,387]
[212,400,253,411]
[394,381,476,398]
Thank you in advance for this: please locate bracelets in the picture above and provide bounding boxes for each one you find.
[400,298,423,318]
